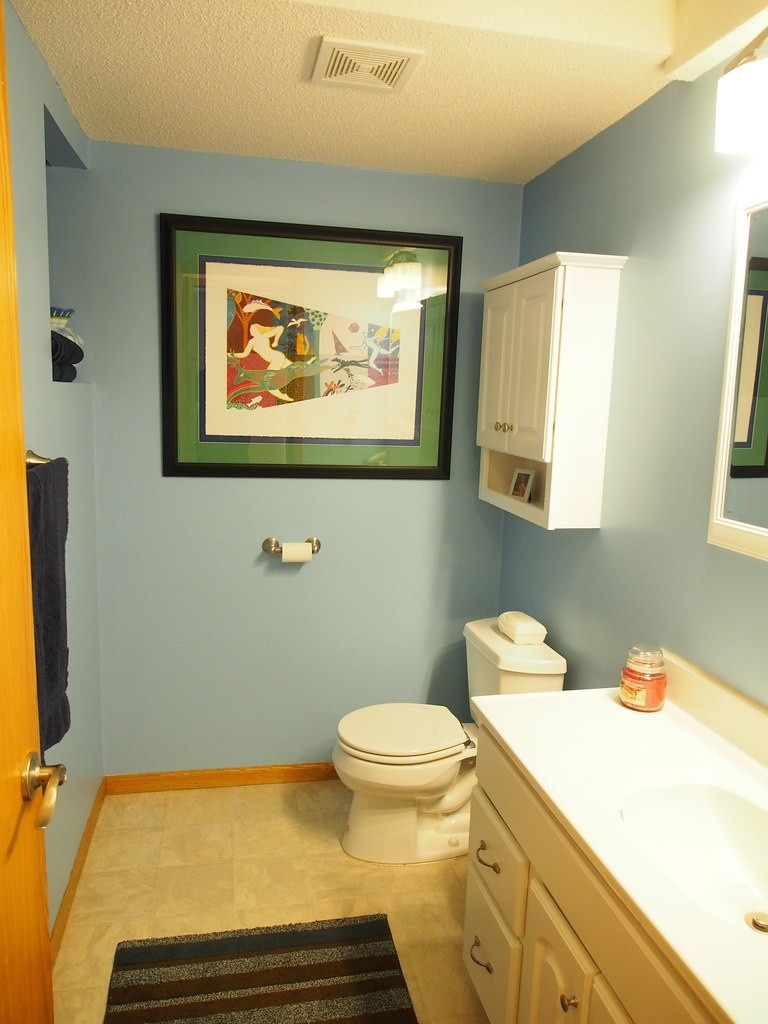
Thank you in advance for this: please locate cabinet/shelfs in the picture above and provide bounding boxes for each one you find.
[478,250,628,526]
[462,725,711,1024]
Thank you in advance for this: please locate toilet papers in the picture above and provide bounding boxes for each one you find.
[281,542,313,562]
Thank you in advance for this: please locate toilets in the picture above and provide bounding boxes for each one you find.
[330,615,568,865]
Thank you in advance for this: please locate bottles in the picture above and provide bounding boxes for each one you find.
[620,644,667,712]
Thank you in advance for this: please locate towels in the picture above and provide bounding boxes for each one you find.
[51,329,84,382]
[27,457,69,749]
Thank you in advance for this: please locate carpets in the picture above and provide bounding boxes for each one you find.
[102,914,419,1024]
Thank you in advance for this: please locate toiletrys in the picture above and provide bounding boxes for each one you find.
[618,646,670,711]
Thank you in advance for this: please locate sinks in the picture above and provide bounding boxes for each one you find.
[589,781,766,972]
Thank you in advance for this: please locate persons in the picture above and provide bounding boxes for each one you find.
[513,474,527,496]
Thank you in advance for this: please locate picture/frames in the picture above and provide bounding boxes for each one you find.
[159,211,463,479]
[733,289,768,449]
[508,467,535,504]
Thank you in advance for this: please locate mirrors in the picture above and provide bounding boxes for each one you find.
[706,200,768,560]
